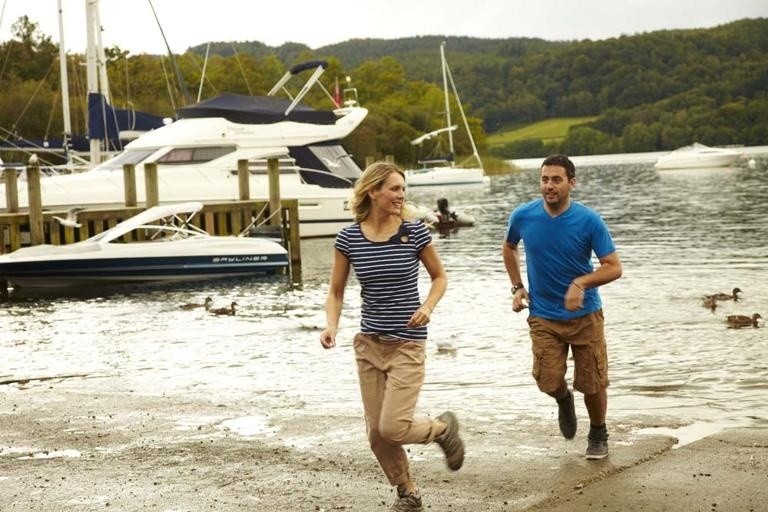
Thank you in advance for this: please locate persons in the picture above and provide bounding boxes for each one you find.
[500,152,626,462]
[318,160,466,512]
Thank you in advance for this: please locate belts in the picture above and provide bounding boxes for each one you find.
[368,334,403,344]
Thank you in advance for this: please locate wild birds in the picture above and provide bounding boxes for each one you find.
[411,125,458,146]
[31,46,42,53]
[28,153,39,164]
[320,155,354,169]
[52,207,88,227]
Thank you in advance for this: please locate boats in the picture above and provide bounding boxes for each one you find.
[656,144,738,167]
[0,202,291,293]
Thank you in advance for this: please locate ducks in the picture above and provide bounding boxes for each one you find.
[436,334,458,351]
[703,288,743,307]
[726,314,764,325]
[178,297,239,316]
[287,280,302,289]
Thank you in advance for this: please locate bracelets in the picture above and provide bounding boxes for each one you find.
[570,281,586,292]
[511,282,524,295]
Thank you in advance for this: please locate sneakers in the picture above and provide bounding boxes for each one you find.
[386,488,422,512]
[433,411,464,470]
[556,389,577,438]
[585,423,609,459]
